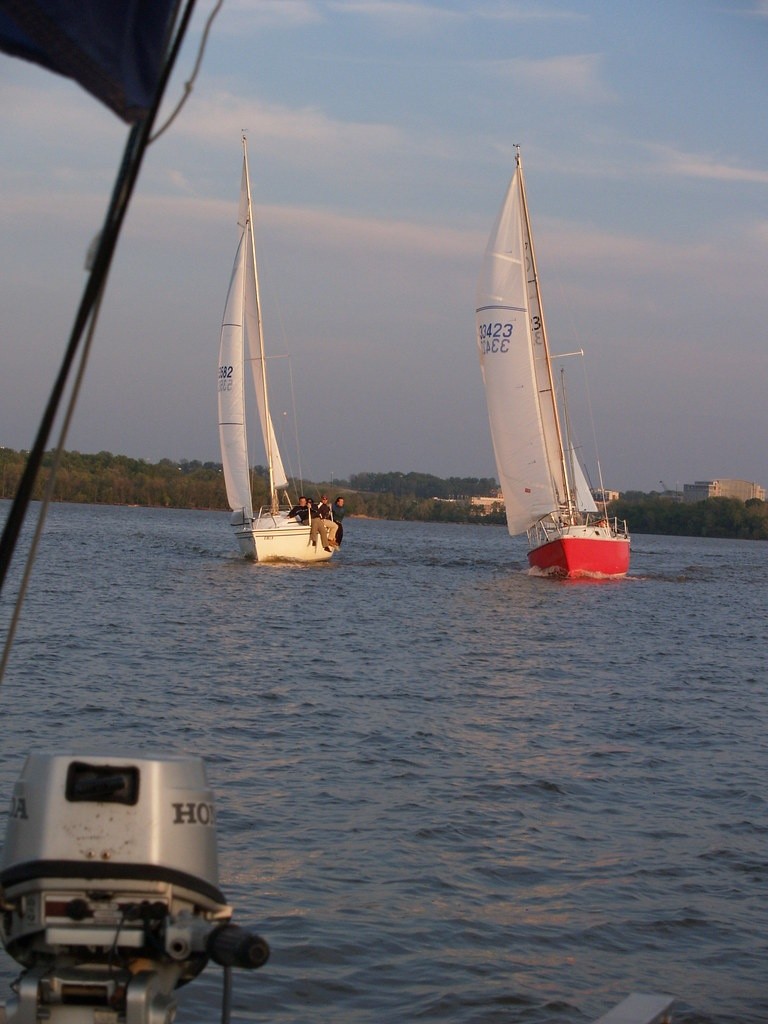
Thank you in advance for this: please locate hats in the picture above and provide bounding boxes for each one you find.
[321,495,328,498]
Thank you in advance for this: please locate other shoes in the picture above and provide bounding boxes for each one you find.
[324,548,332,552]
[328,539,337,547]
[313,541,317,546]
[336,546,340,552]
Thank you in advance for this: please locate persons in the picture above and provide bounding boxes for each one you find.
[285,495,345,552]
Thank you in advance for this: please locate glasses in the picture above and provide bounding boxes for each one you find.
[323,499,328,500]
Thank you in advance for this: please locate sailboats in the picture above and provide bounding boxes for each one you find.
[473,141,632,579]
[215,126,344,565]
